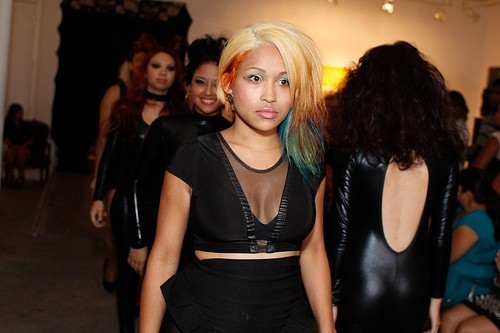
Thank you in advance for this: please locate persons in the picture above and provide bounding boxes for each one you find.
[3,104,35,187]
[90,34,226,332]
[138,21,335,333]
[323,40,461,333]
[438,78,500,332]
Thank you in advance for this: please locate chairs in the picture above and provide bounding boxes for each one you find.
[3,121,51,185]
